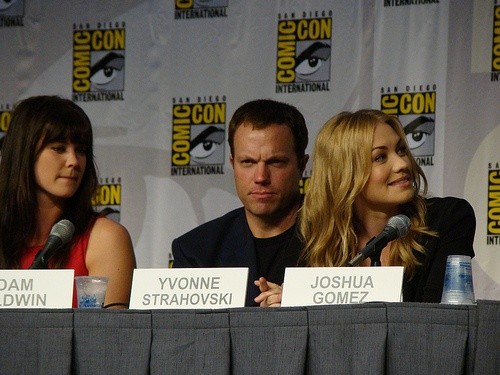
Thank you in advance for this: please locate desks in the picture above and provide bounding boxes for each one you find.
[0,301,500,375]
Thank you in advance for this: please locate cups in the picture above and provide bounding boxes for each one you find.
[440,255,477,305]
[74,276,108,309]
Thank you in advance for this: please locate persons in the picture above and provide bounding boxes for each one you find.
[0,96,137,309]
[254,109,476,306]
[171,100,308,307]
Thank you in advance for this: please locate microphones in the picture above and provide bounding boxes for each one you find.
[28,219,75,270]
[347,214,411,268]
